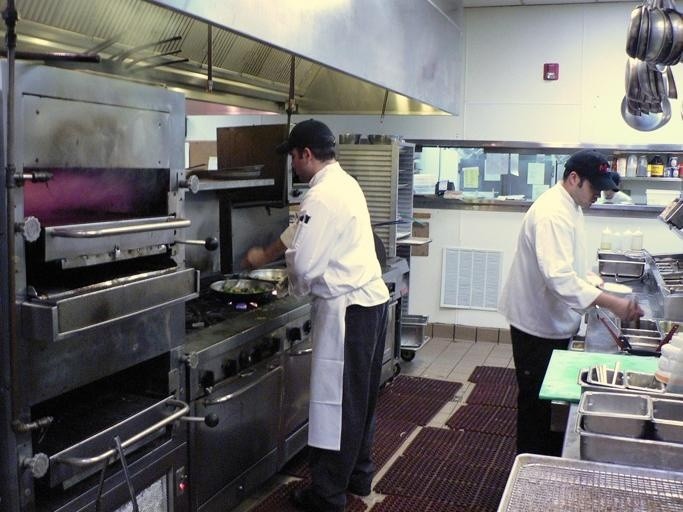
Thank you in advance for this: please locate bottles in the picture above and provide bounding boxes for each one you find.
[627,153,664,177]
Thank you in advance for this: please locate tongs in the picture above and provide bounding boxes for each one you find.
[93,435,140,511]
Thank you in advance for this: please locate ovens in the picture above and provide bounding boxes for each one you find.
[0,54,201,509]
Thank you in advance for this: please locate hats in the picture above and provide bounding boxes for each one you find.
[273,116,338,157]
[563,147,620,197]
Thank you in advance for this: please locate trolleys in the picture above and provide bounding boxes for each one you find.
[401,315,430,361]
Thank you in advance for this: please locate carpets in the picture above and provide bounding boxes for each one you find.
[252,365,519,512]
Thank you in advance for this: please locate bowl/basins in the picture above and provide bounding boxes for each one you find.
[597,281,633,297]
[367,134,400,145]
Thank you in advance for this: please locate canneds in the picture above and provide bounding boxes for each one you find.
[664,156,680,178]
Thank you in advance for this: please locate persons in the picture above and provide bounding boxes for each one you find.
[239,119,389,510]
[595,169,636,204]
[493,149,643,463]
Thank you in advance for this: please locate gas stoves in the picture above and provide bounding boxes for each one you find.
[185,286,284,323]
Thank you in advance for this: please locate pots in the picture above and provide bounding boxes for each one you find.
[211,278,277,301]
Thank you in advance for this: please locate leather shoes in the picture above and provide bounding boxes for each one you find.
[283,478,372,512]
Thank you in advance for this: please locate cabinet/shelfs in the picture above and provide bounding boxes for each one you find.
[336,142,432,376]
[405,138,683,212]
